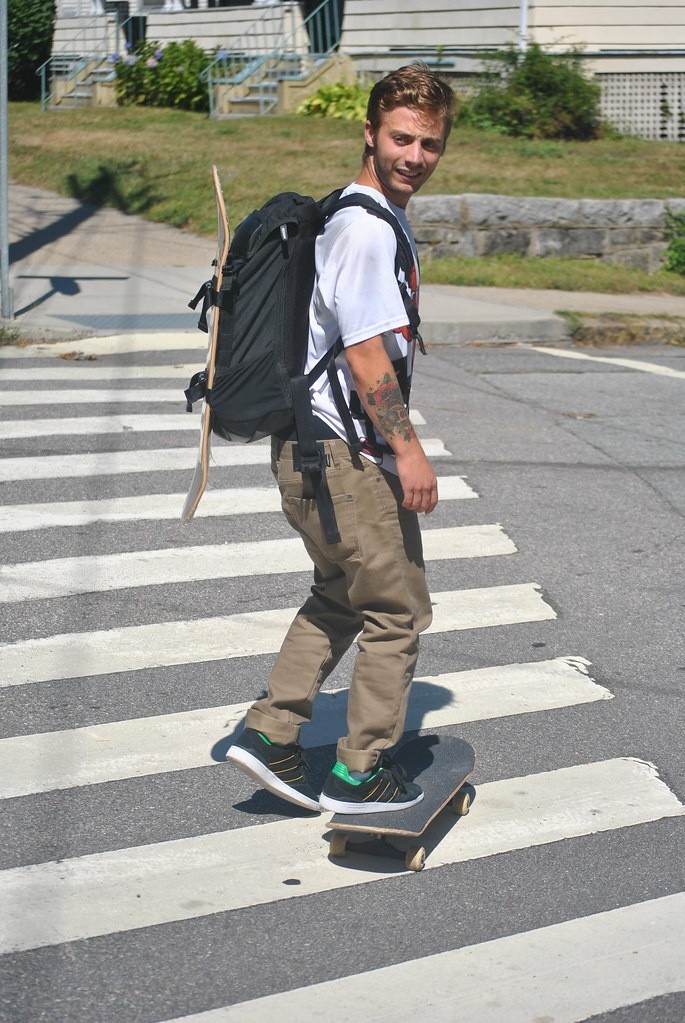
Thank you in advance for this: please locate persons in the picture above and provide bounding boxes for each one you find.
[225,64,455,814]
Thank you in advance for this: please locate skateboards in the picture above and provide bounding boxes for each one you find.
[325,734,476,871]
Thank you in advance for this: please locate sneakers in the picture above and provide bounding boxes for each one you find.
[226,728,322,813]
[319,747,424,815]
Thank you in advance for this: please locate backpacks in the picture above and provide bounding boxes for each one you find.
[185,187,420,443]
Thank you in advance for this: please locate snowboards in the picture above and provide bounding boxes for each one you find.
[180,166,232,523]
